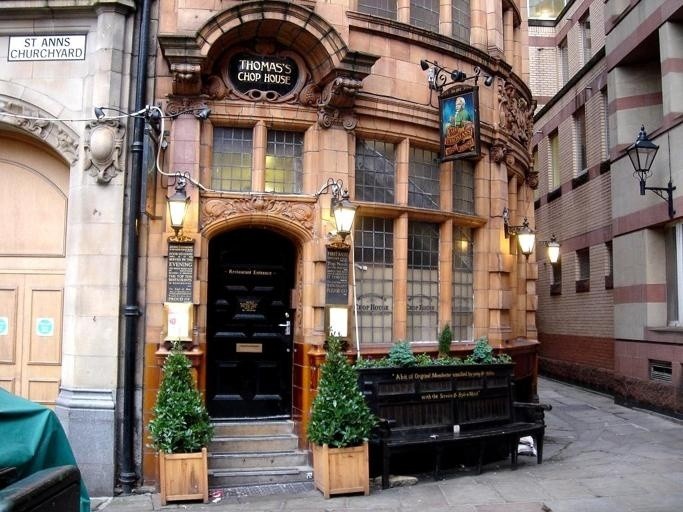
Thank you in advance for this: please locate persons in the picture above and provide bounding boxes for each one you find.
[451,96,470,127]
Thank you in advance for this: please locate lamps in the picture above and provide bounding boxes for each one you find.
[506,218,536,263]
[167,175,191,236]
[625,125,676,220]
[333,189,358,242]
[545,234,563,267]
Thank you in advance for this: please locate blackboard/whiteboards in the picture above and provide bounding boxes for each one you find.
[325,249,350,305]
[166,243,194,302]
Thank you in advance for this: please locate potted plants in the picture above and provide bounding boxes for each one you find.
[146,339,213,507]
[305,333,380,499]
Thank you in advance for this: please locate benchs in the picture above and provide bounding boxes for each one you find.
[356,362,552,489]
